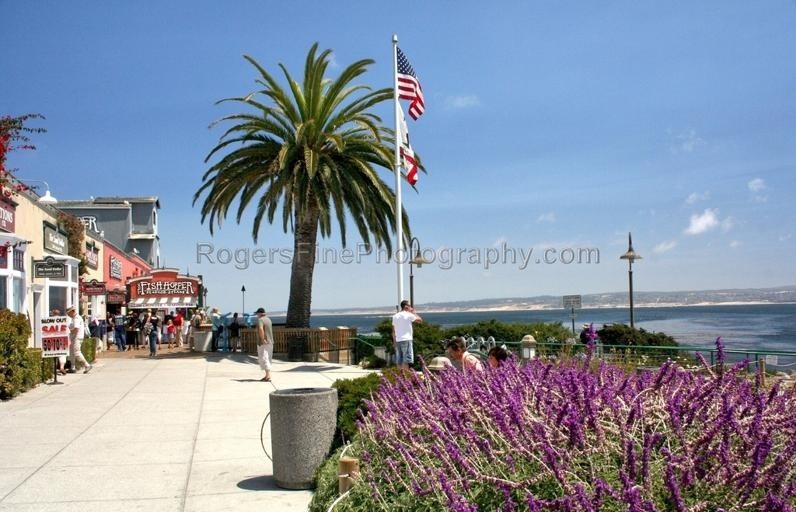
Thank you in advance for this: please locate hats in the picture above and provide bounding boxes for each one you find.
[255,308,265,313]
[66,307,75,314]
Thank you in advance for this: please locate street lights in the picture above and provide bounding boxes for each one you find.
[409,236,431,306]
[240,285,246,316]
[620,232,643,326]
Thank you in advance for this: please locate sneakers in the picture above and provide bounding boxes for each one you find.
[84,365,91,373]
[60,369,75,374]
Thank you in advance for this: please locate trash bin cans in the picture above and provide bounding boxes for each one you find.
[192,324,213,351]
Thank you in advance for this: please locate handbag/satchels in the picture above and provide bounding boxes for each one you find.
[143,323,153,336]
[218,326,223,333]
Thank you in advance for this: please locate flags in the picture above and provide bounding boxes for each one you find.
[398,102,419,186]
[397,46,427,120]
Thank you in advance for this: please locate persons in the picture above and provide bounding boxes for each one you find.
[488,347,507,367]
[254,308,274,382]
[392,300,422,370]
[580,323,590,344]
[54,306,241,375]
[446,338,482,372]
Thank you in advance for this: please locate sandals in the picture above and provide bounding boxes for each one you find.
[261,377,271,382]
